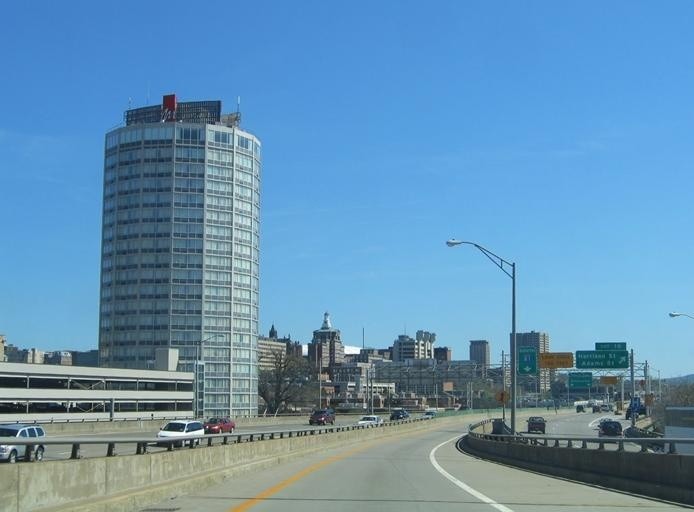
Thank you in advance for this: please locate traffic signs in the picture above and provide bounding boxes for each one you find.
[576,349,630,369]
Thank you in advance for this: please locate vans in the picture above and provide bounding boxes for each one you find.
[156,420,205,446]
[0,424,46,464]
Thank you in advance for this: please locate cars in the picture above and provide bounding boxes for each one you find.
[626,407,640,420]
[357,415,385,426]
[309,410,336,425]
[202,417,236,434]
[390,410,410,421]
[576,402,613,413]
[598,421,622,443]
[527,416,547,434]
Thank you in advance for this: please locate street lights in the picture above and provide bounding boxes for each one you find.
[319,353,334,410]
[195,334,226,419]
[446,238,517,438]
